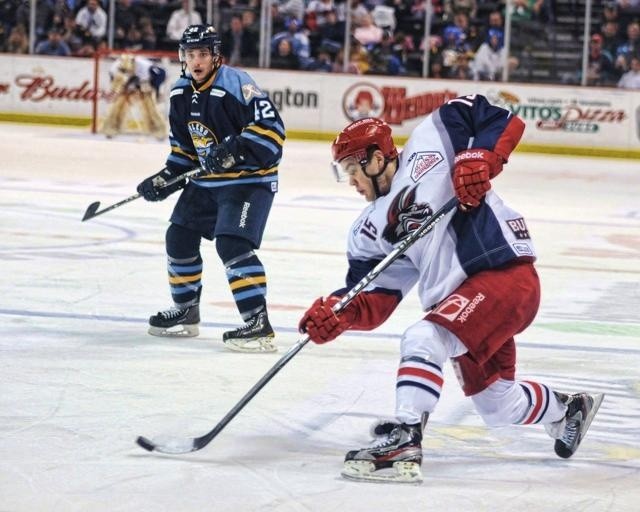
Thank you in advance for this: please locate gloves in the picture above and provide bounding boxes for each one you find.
[204,145,237,171]
[136,177,173,201]
[297,296,358,344]
[452,148,503,212]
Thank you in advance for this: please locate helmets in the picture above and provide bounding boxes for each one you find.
[331,120,396,171]
[177,22,223,63]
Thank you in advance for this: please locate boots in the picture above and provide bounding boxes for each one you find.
[555,392,593,458]
[222,308,275,339]
[342,425,426,464]
[148,308,200,326]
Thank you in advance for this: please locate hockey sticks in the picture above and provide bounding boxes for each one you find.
[83,155,235,220]
[137,198,457,456]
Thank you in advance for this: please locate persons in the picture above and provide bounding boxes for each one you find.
[135,22,287,338]
[299,94,594,464]
[101,56,168,140]
